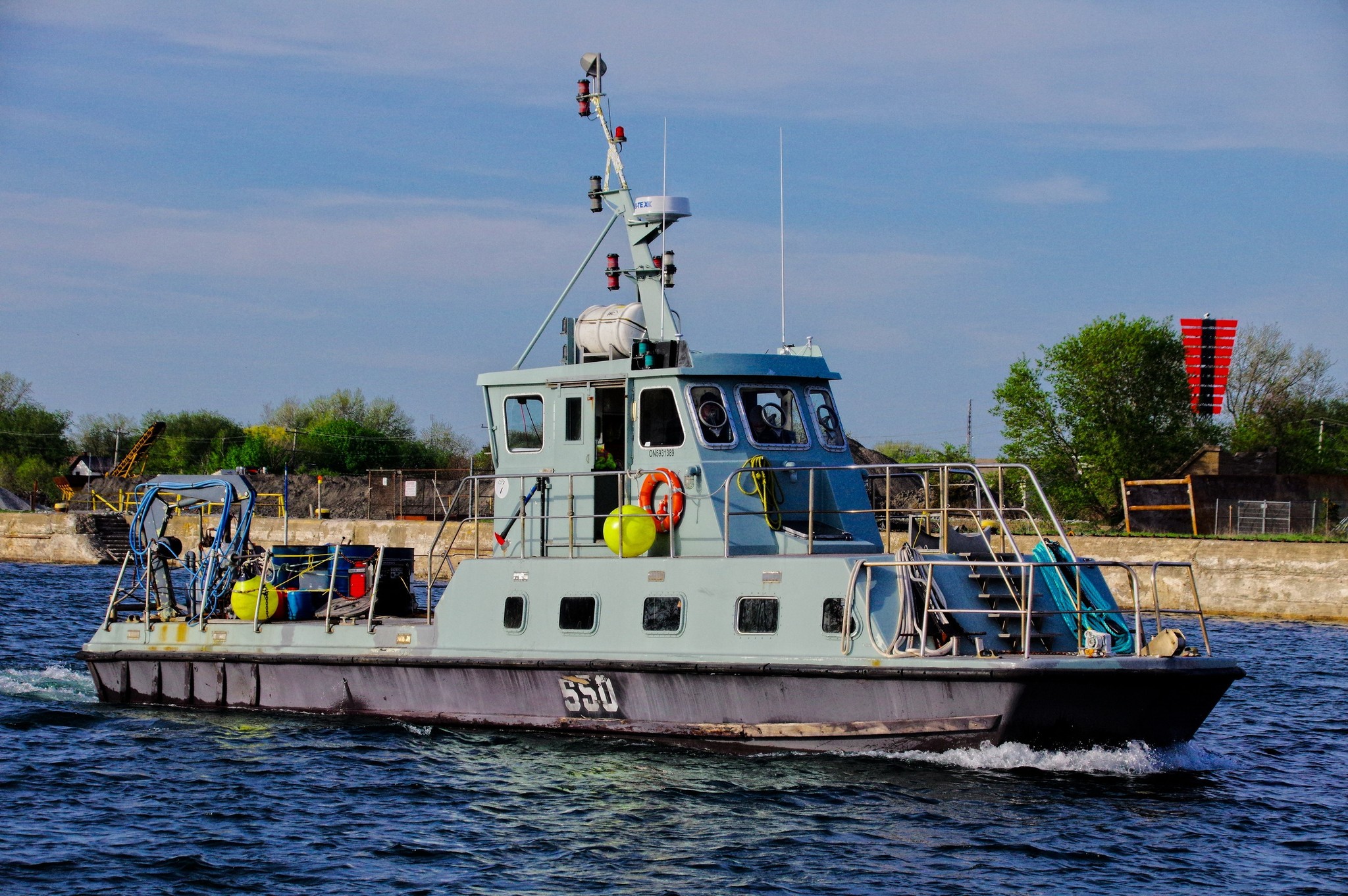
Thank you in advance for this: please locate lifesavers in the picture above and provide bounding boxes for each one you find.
[639,468,683,534]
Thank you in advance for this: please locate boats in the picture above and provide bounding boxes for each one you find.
[72,49,1251,756]
